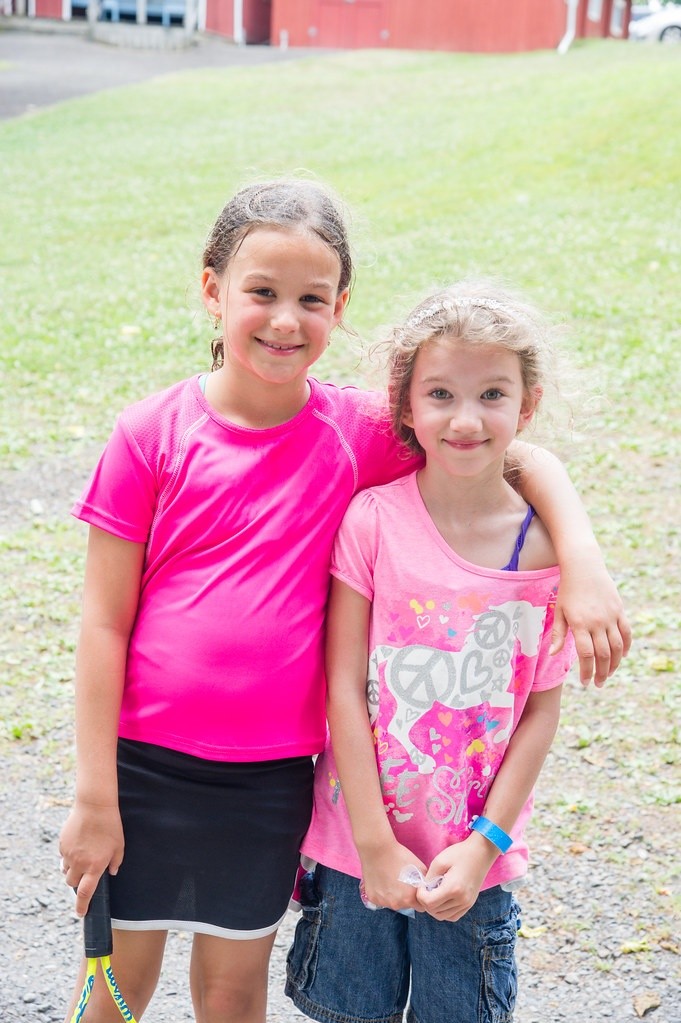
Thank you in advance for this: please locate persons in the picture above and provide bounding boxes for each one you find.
[60,179,631,1023]
[282,285,576,1023]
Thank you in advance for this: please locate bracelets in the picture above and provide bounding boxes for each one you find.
[470,815,513,856]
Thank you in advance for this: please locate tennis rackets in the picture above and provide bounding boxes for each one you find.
[65,864,139,1023]
